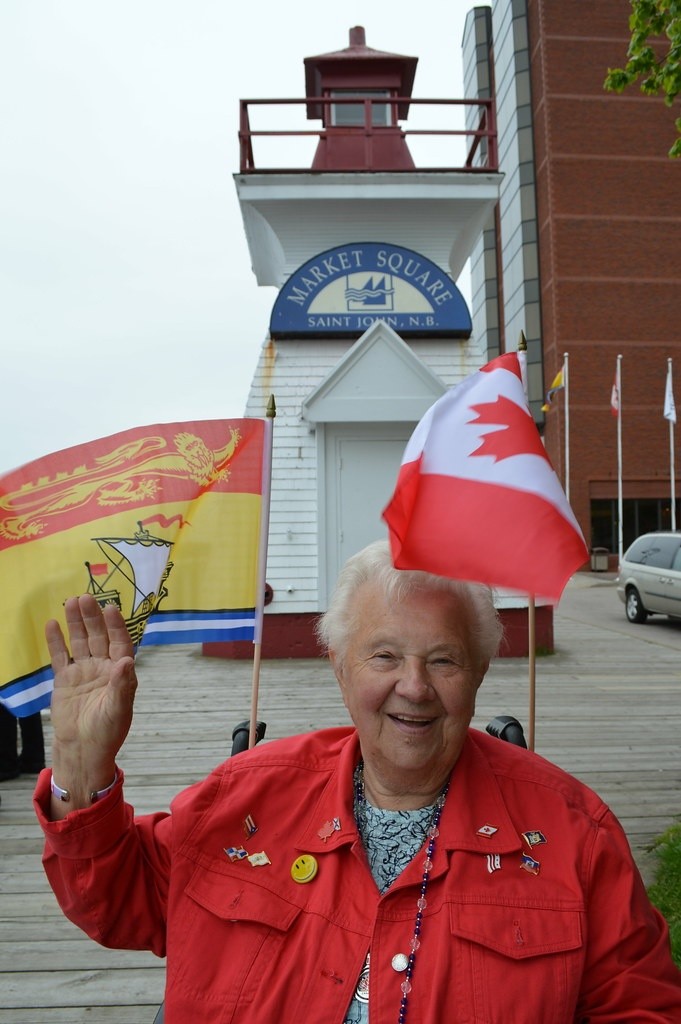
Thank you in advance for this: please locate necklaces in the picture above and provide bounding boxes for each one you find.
[356,761,449,1024]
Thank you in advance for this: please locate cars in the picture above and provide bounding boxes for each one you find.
[614,531,680,625]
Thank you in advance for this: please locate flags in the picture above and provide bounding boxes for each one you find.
[610,367,620,420]
[379,353,590,607]
[663,368,676,424]
[0,417,267,718]
[540,364,565,412]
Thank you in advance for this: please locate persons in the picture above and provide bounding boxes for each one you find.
[32,539,680,1024]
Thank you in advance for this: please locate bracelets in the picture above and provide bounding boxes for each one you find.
[51,770,118,802]
